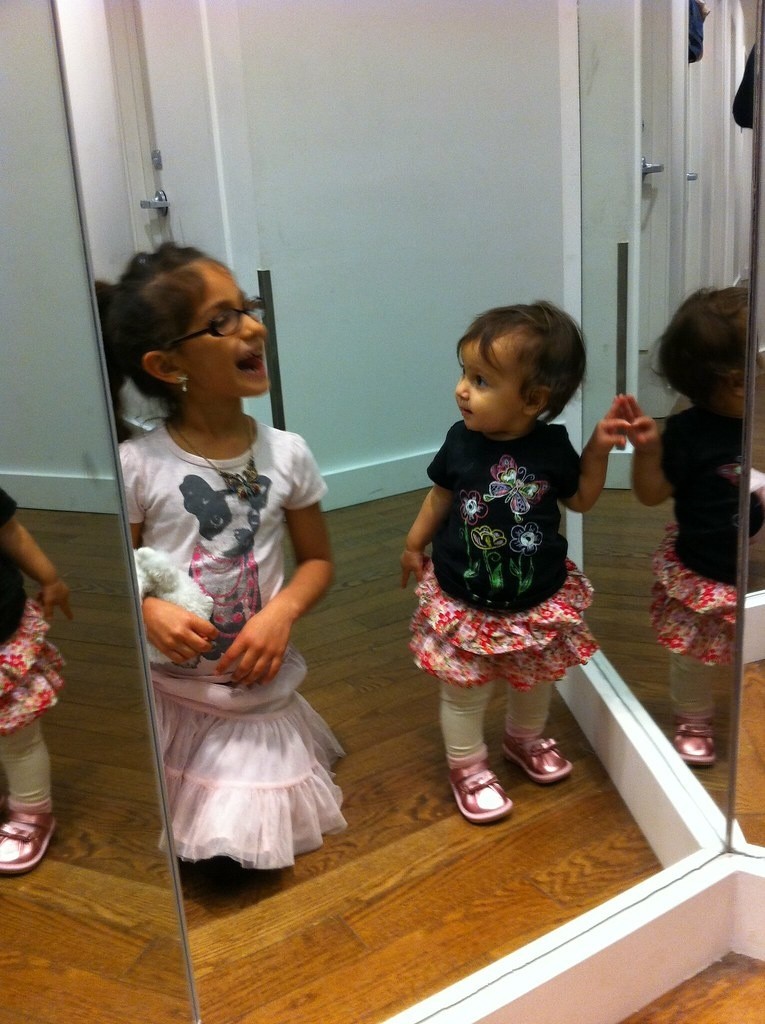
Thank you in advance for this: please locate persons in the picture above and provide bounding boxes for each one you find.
[732,44,755,130]
[0,488,75,875]
[93,240,348,868]
[399,300,630,823]
[631,287,760,765]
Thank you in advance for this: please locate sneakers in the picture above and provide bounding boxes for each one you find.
[673,715,716,765]
[451,760,513,823]
[0,811,56,874]
[502,729,571,783]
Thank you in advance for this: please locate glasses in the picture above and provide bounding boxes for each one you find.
[160,294,267,352]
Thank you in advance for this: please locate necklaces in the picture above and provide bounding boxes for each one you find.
[174,417,260,499]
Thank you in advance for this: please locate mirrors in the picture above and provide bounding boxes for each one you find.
[0,0,765,1024]
